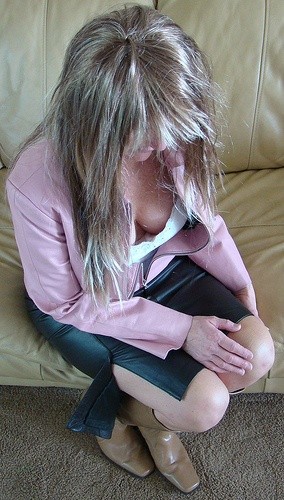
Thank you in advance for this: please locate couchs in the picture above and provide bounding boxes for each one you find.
[0,0,284,393]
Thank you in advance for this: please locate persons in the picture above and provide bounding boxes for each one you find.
[6,3,274,494]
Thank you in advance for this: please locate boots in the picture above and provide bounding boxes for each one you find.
[127,387,248,494]
[94,402,182,478]
